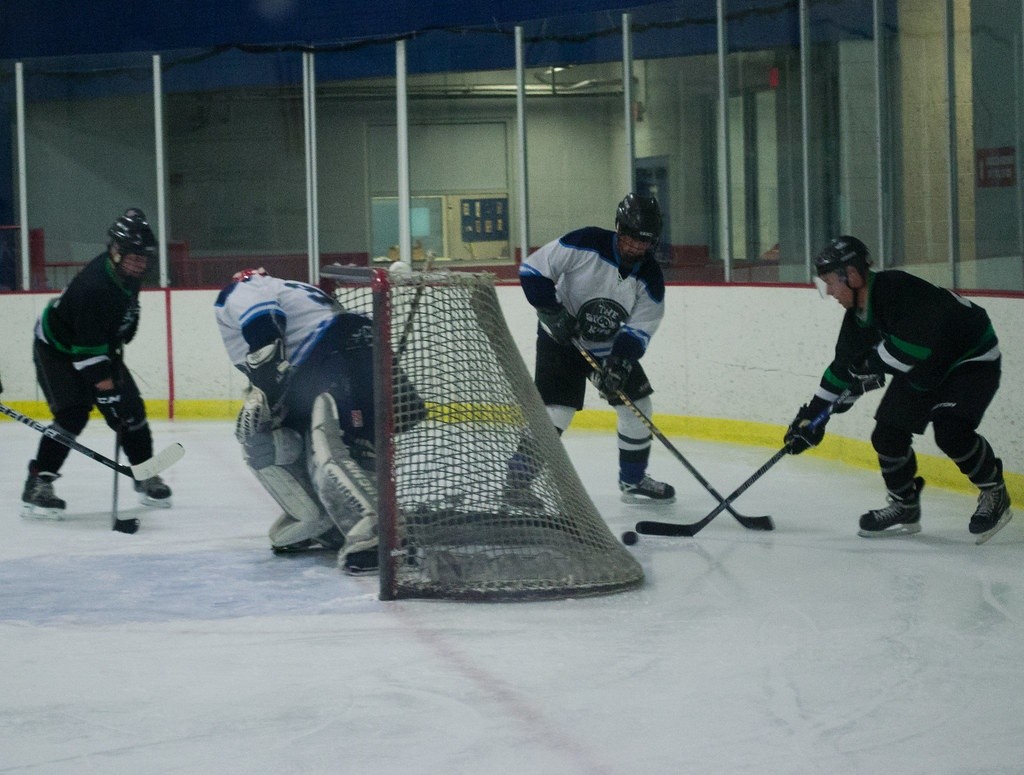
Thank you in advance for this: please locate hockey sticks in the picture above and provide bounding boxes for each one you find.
[395,249,438,360]
[0,404,185,481]
[112,431,141,536]
[635,388,852,537]
[568,336,775,532]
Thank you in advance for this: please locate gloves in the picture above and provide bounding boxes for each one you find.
[847,344,886,396]
[117,305,140,345]
[597,357,632,402]
[536,304,578,346]
[784,401,830,455]
[95,388,134,434]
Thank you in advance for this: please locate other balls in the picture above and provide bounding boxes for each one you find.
[622,532,637,546]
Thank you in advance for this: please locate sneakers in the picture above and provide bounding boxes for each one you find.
[968,458,1013,544]
[19,459,67,520]
[500,485,547,517]
[134,475,172,508]
[857,476,925,538]
[345,538,419,575]
[270,525,345,554]
[618,469,677,506]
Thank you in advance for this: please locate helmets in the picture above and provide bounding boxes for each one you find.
[231,268,268,282]
[108,216,157,257]
[816,233,873,278]
[611,193,664,269]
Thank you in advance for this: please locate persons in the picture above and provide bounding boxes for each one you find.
[503,193,675,514]
[784,235,1012,545]
[212,268,428,574]
[19,209,173,521]
